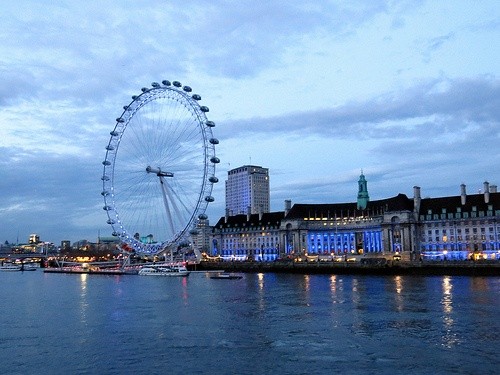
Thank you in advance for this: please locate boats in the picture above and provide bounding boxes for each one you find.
[18,261,40,271]
[138,264,192,277]
[0,262,21,271]
[210,272,244,281]
